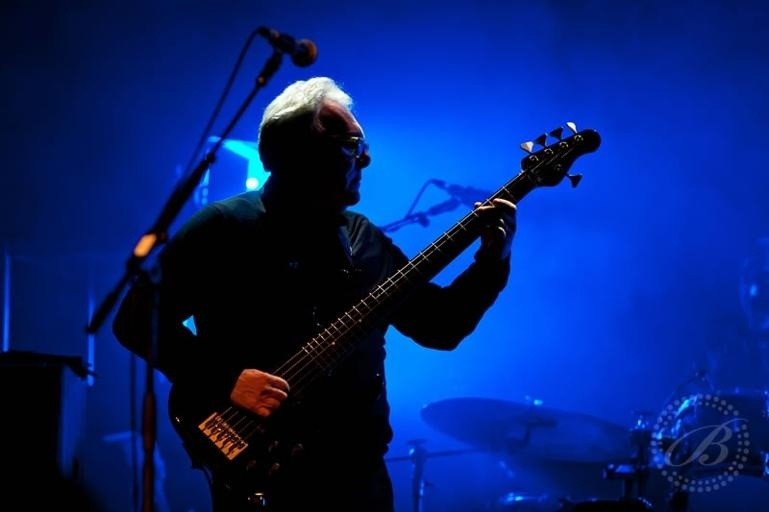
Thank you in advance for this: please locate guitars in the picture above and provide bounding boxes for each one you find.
[169,121,600,476]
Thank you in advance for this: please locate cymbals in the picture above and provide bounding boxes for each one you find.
[421,400,633,457]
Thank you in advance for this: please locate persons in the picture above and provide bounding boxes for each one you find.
[112,76,517,512]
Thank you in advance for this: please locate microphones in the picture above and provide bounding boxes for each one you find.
[258,26,318,67]
[432,177,494,206]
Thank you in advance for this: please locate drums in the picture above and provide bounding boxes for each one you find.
[670,396,767,512]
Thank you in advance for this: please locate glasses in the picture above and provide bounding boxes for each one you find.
[338,136,369,158]
